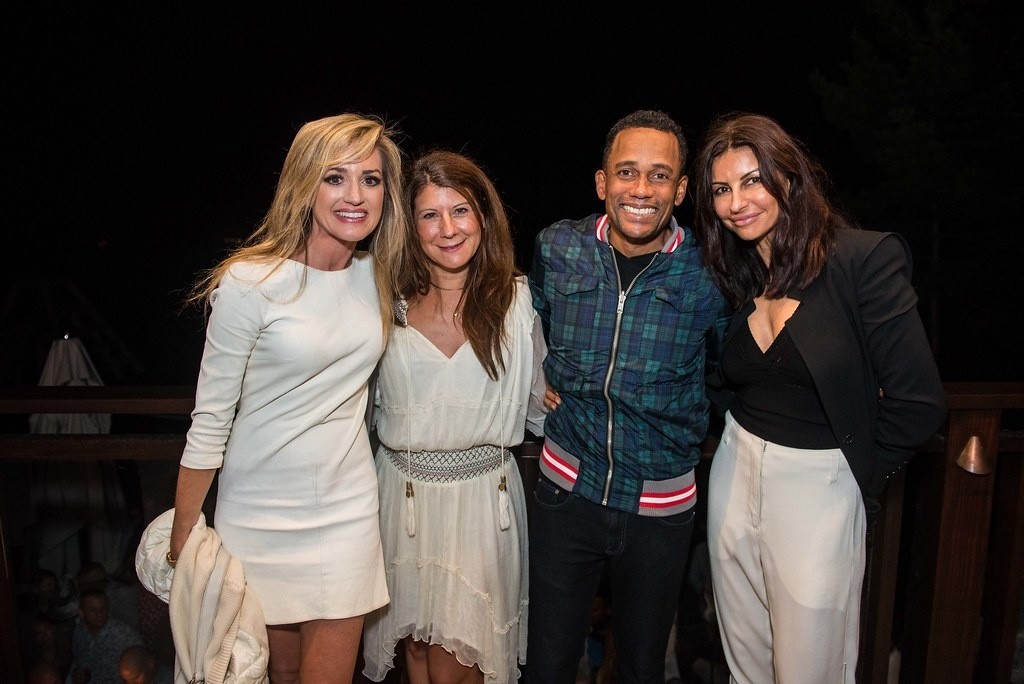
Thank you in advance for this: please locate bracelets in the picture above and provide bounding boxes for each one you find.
[166,552,178,569]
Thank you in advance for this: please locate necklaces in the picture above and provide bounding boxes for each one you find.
[443,300,463,317]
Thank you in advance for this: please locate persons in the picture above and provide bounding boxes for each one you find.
[167,113,562,684]
[362,150,550,684]
[22,561,176,684]
[515,111,732,684]
[544,116,951,684]
